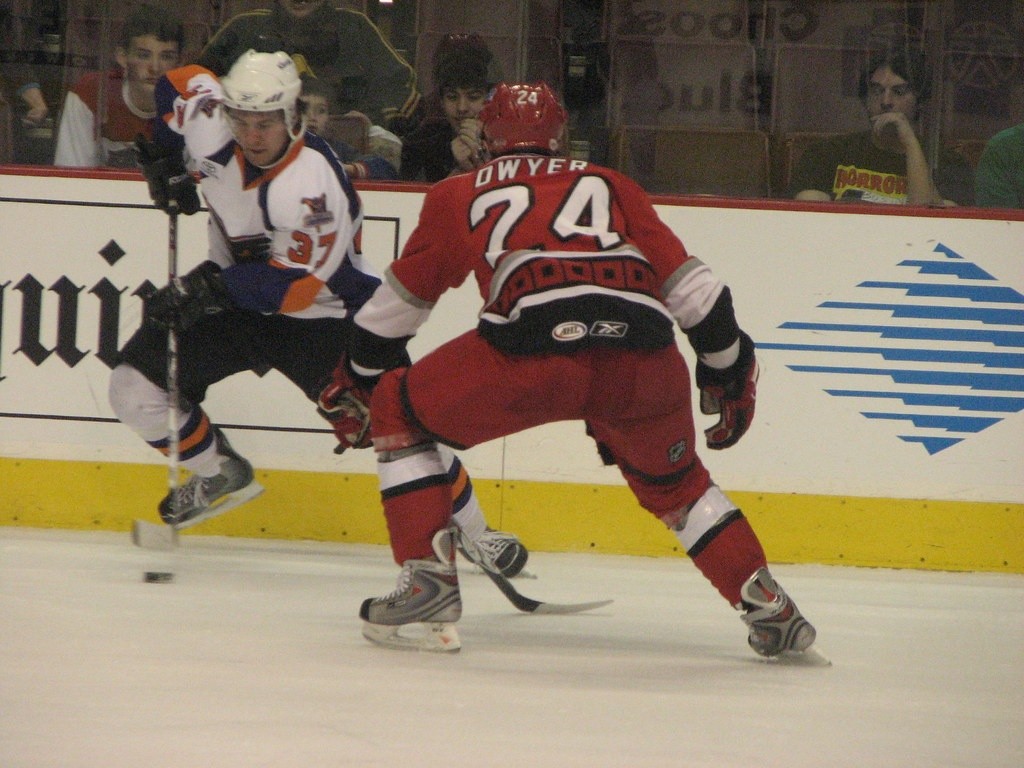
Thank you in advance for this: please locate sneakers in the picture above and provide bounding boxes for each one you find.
[357,528,464,650]
[158,422,263,530]
[455,527,539,581]
[735,567,833,668]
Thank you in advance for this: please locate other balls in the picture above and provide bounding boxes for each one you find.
[144,571,176,582]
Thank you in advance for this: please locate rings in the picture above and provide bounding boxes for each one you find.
[478,145,483,152]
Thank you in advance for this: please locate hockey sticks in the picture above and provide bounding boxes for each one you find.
[131,198,181,553]
[453,515,614,616]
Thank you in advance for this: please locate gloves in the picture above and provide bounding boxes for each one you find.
[316,355,378,449]
[135,133,202,215]
[695,330,760,451]
[146,261,230,328]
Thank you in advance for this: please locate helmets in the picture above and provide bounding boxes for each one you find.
[218,47,303,111]
[479,81,569,159]
[435,31,482,63]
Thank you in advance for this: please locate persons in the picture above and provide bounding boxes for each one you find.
[397,53,491,184]
[973,123,1023,209]
[196,0,427,133]
[15,20,63,120]
[300,77,398,180]
[53,4,188,169]
[316,82,814,657]
[789,46,949,206]
[110,43,542,578]
